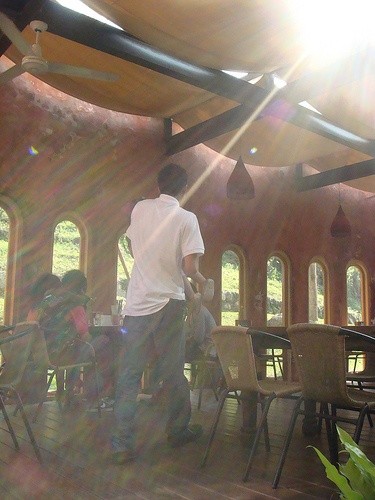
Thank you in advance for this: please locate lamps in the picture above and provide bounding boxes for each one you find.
[226,130,254,200]
[330,183,351,237]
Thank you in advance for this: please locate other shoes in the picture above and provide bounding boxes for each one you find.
[171,424,201,448]
[113,446,136,464]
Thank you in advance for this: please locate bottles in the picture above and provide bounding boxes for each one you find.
[250,320,254,330]
[118,300,122,313]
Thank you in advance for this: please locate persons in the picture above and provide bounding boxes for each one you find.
[150,283,217,404]
[27,270,112,410]
[112,164,206,466]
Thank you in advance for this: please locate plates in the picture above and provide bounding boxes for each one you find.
[95,325,112,327]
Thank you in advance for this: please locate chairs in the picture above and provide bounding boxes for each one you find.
[0,321,102,464]
[182,323,375,490]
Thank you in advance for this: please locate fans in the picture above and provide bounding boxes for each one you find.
[0,10,121,85]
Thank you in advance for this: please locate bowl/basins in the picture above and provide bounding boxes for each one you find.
[101,313,112,324]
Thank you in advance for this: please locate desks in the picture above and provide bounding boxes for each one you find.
[237,325,375,450]
[89,324,121,418]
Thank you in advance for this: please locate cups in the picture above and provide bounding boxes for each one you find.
[113,314,121,326]
